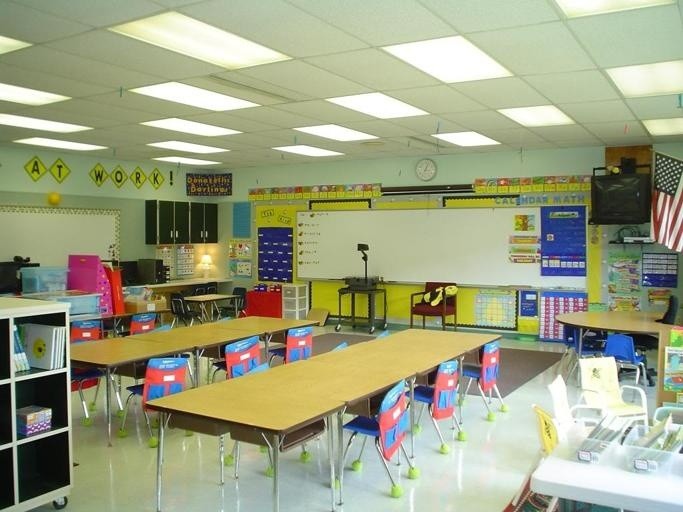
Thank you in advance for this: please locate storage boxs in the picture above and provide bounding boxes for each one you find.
[123,300,167,313]
[53,293,102,316]
[19,266,71,295]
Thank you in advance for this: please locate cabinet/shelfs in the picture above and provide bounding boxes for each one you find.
[0,297,72,511]
[145,199,190,244]
[186,200,218,245]
[282,282,310,320]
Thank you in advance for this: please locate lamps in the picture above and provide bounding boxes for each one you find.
[195,254,216,279]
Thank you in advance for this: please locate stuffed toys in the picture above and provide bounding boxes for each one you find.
[420,285,458,306]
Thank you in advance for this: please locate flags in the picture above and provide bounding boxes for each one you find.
[648,151,682,252]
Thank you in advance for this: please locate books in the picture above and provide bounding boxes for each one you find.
[124,286,164,303]
[569,411,682,475]
[12,323,66,374]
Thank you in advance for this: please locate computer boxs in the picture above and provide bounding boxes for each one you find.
[137,259,163,285]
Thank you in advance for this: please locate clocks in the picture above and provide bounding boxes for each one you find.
[414,157,438,181]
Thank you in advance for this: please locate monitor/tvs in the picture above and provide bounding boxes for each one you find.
[119,261,137,284]
[0,262,40,294]
[591,174,651,224]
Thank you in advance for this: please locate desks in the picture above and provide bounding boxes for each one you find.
[145,326,503,511]
[335,288,387,334]
[70,314,319,448]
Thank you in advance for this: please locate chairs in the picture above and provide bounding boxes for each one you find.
[554,295,678,389]
[456,340,508,422]
[69,319,124,420]
[128,314,157,333]
[405,358,467,455]
[168,281,249,329]
[329,379,421,499]
[212,336,265,384]
[411,281,457,332]
[119,359,189,447]
[267,327,314,366]
[507,349,682,512]
